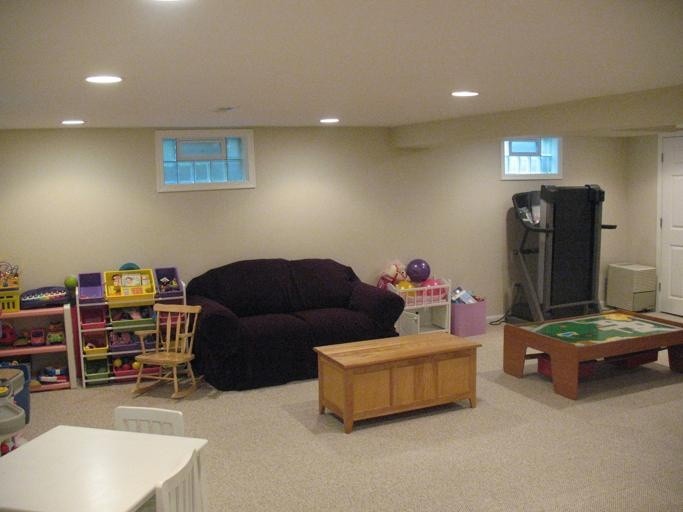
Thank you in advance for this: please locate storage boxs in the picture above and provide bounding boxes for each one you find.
[78,268,184,386]
[451,298,486,337]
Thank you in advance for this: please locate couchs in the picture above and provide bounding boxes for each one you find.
[186,259,405,392]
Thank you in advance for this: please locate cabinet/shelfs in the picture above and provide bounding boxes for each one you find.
[605,264,656,312]
[0,303,76,392]
[386,281,451,337]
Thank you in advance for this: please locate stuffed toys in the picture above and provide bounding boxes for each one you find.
[376,263,411,293]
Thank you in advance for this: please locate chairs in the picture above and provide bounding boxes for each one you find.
[157,448,203,512]
[132,303,203,398]
[115,406,183,435]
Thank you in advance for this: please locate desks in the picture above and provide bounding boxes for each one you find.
[0,426,210,511]
[504,308,682,400]
